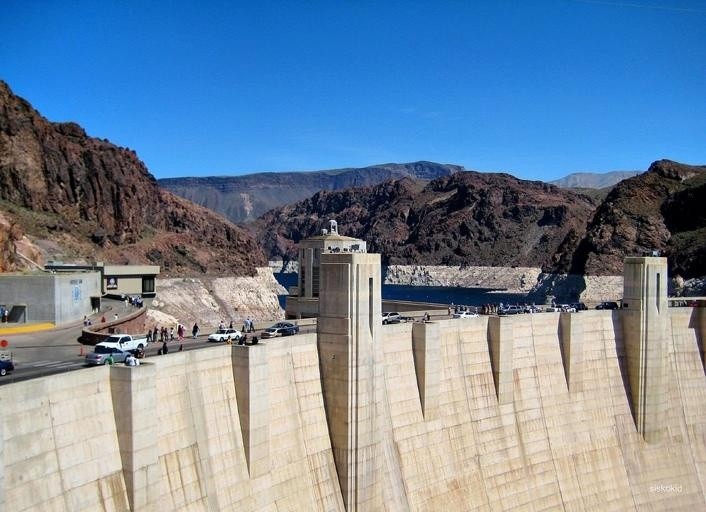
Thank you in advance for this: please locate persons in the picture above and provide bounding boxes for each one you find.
[421,301,542,323]
[119,291,144,308]
[217,317,259,345]
[87,318,91,326]
[113,313,119,320]
[162,342,169,354]
[82,315,87,326]
[146,322,200,341]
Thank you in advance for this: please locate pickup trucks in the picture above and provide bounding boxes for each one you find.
[95,333,148,352]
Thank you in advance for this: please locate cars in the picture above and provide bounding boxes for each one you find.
[265,322,299,335]
[546,302,589,313]
[208,329,242,342]
[452,311,479,320]
[524,305,542,312]
[84,347,131,365]
[262,328,293,338]
[0,360,14,376]
[382,312,400,325]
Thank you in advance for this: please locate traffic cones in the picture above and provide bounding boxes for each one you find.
[78,344,86,357]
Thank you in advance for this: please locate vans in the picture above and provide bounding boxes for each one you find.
[595,301,619,310]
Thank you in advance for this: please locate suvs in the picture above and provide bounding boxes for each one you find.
[497,305,522,316]
